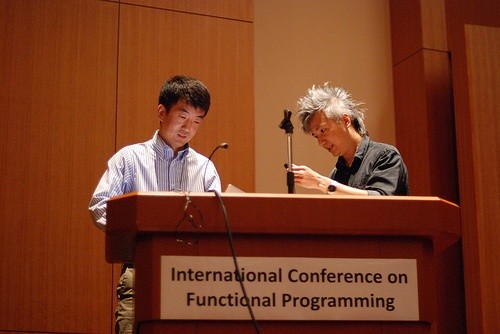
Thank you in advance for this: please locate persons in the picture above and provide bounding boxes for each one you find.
[287,81,409,196]
[88,74,220,334]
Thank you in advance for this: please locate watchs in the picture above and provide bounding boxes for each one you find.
[326,179,338,195]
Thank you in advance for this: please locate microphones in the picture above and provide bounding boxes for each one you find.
[203,143,228,191]
[326,185,336,194]
